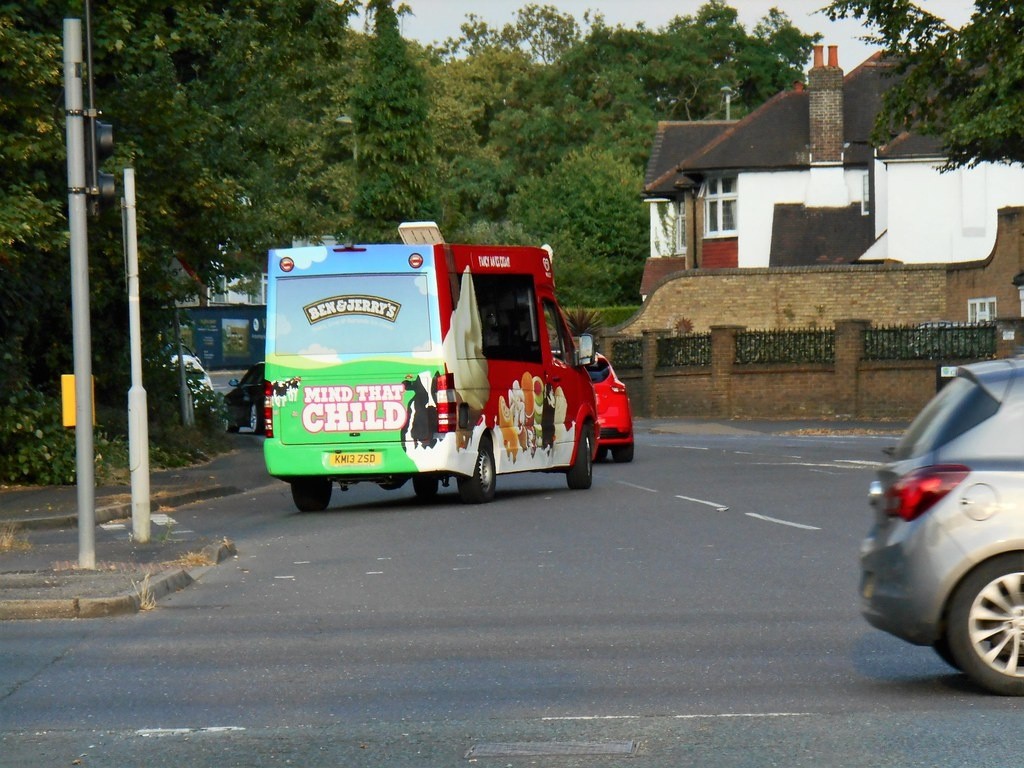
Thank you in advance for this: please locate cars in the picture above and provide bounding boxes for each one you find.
[583,351,634,464]
[220,362,266,435]
[167,341,215,409]
[857,352,1024,699]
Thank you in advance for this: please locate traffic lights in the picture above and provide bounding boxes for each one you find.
[84,116,115,222]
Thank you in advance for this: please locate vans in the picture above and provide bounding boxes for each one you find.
[264,221,598,512]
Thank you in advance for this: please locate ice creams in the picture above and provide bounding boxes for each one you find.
[439,264,493,449]
